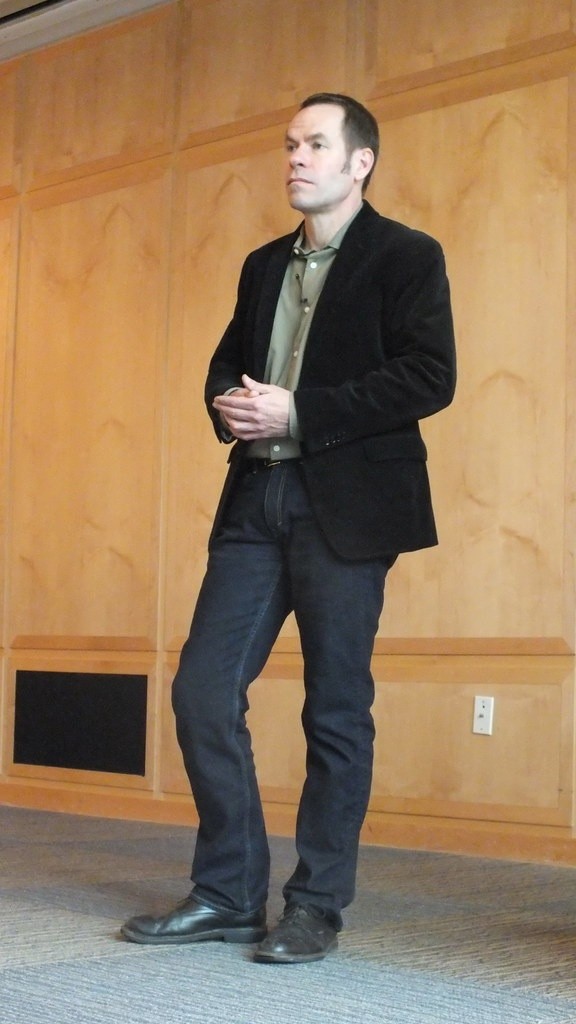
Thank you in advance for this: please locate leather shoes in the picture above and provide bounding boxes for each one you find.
[253,894,340,963]
[121,892,267,946]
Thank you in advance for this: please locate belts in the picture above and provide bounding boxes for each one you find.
[251,454,296,468]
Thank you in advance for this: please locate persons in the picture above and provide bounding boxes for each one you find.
[117,94,455,964]
